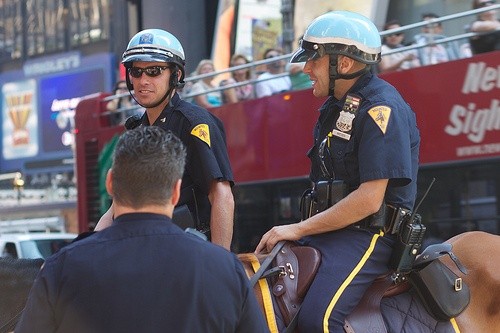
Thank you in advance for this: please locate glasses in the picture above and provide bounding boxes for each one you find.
[128,65,170,77]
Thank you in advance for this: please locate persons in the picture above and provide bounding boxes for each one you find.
[10,168,76,200]
[93,28,236,250]
[8,126,273,333]
[254,11,422,333]
[107,0,500,123]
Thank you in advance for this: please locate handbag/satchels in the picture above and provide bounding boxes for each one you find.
[409,242,470,322]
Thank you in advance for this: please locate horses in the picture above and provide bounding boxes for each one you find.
[0,230,500,333]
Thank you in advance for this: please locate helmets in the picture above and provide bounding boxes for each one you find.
[300,10,382,63]
[120,28,186,79]
